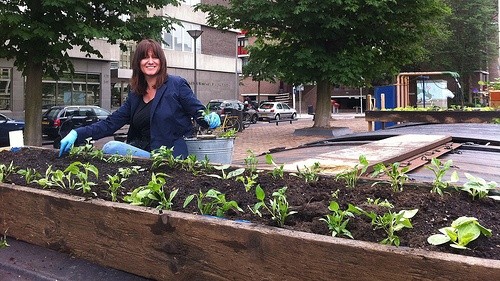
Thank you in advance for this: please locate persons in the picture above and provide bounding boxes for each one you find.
[59,39,221,158]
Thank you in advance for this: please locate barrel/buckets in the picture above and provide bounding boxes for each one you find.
[184,136,235,165]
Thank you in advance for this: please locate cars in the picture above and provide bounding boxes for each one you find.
[0,113,25,147]
[257,102,297,121]
[114,124,130,142]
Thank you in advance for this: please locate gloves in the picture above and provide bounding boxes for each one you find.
[204,112,221,128]
[58,129,78,158]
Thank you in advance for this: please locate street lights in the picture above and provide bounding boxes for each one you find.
[187,29,204,99]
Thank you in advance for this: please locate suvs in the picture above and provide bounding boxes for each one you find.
[42,105,112,141]
[206,98,259,124]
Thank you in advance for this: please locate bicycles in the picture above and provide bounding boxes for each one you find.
[191,110,252,135]
